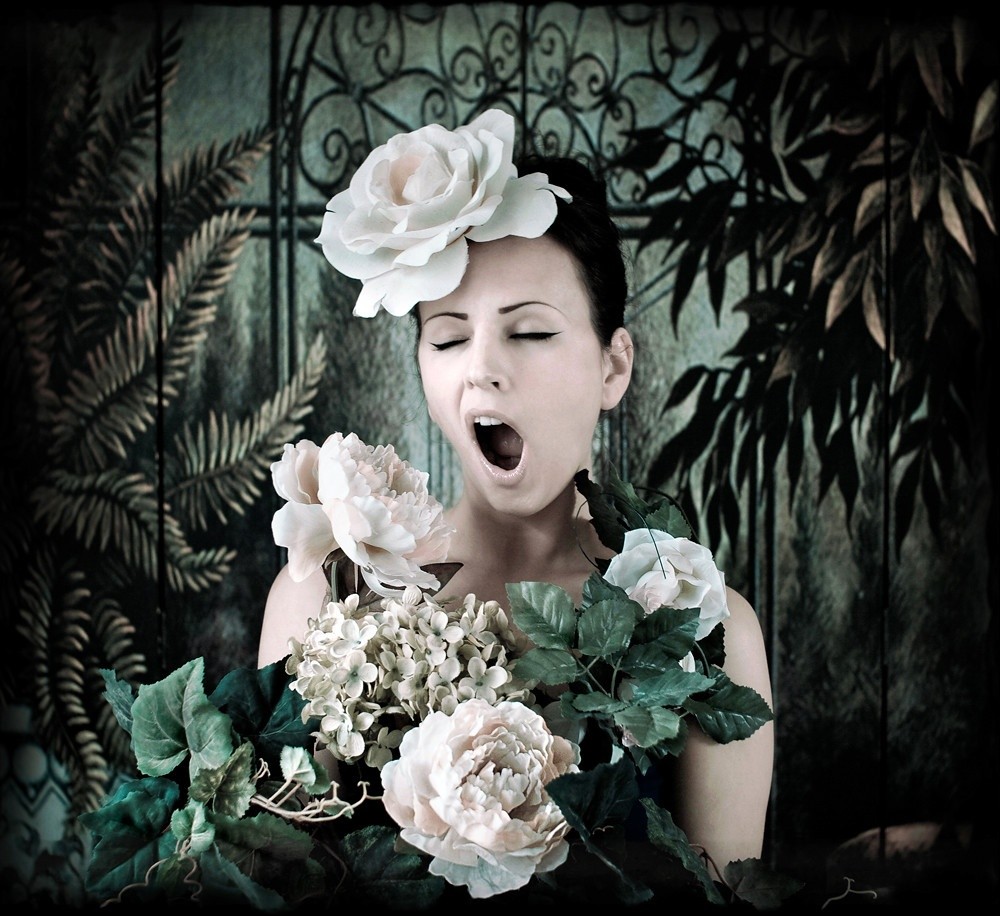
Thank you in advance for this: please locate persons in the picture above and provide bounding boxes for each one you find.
[259,147,775,880]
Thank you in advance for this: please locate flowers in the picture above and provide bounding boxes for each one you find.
[316,107,573,319]
[0,430,805,916]
